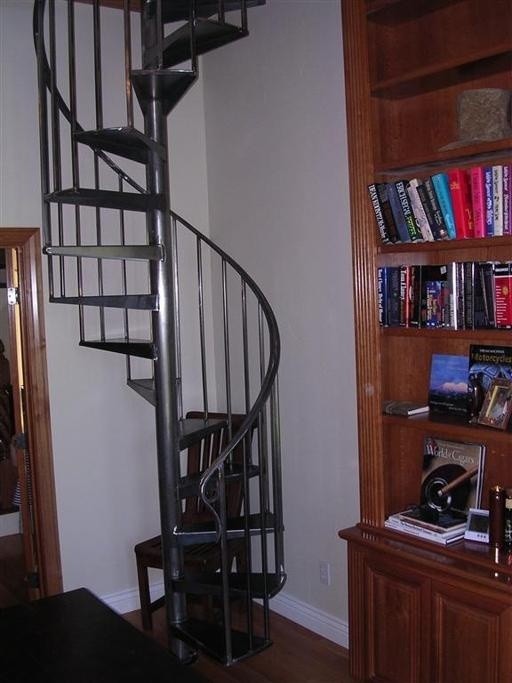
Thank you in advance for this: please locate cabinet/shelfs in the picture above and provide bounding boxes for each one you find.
[334,0,509,683]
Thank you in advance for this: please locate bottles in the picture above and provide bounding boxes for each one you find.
[488,484,506,548]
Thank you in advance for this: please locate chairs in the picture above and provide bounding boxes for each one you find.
[133,398,266,635]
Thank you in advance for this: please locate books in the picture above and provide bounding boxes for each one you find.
[464,343,512,431]
[367,163,512,329]
[427,352,469,422]
[385,435,486,545]
[383,399,430,417]
[503,488,512,556]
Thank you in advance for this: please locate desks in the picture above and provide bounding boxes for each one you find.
[0,583,210,681]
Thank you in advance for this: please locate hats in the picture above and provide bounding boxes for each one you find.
[435,88,512,153]
